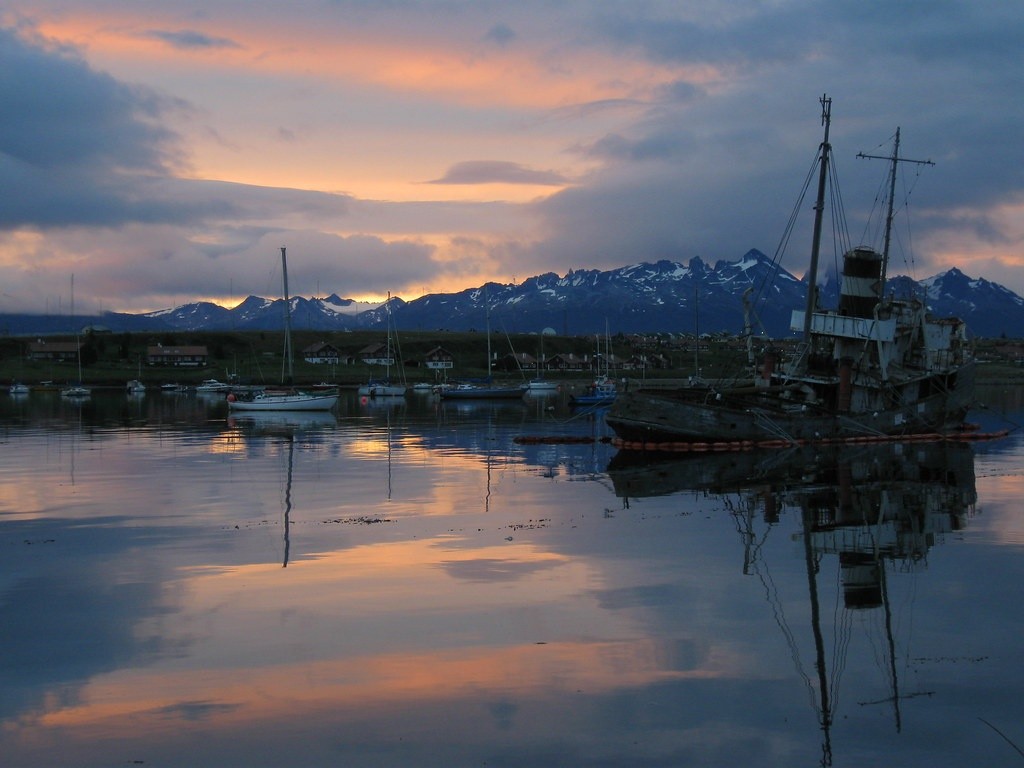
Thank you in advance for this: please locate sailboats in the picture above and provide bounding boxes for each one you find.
[521,335,560,390]
[557,434,980,768]
[356,291,409,397]
[226,408,338,568]
[226,243,341,411]
[515,88,1024,469]
[442,288,532,401]
[558,294,754,430]
[57,272,93,397]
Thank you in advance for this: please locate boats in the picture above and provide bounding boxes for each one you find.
[9,378,31,394]
[160,383,178,391]
[195,378,232,393]
[126,355,147,393]
[411,351,483,398]
